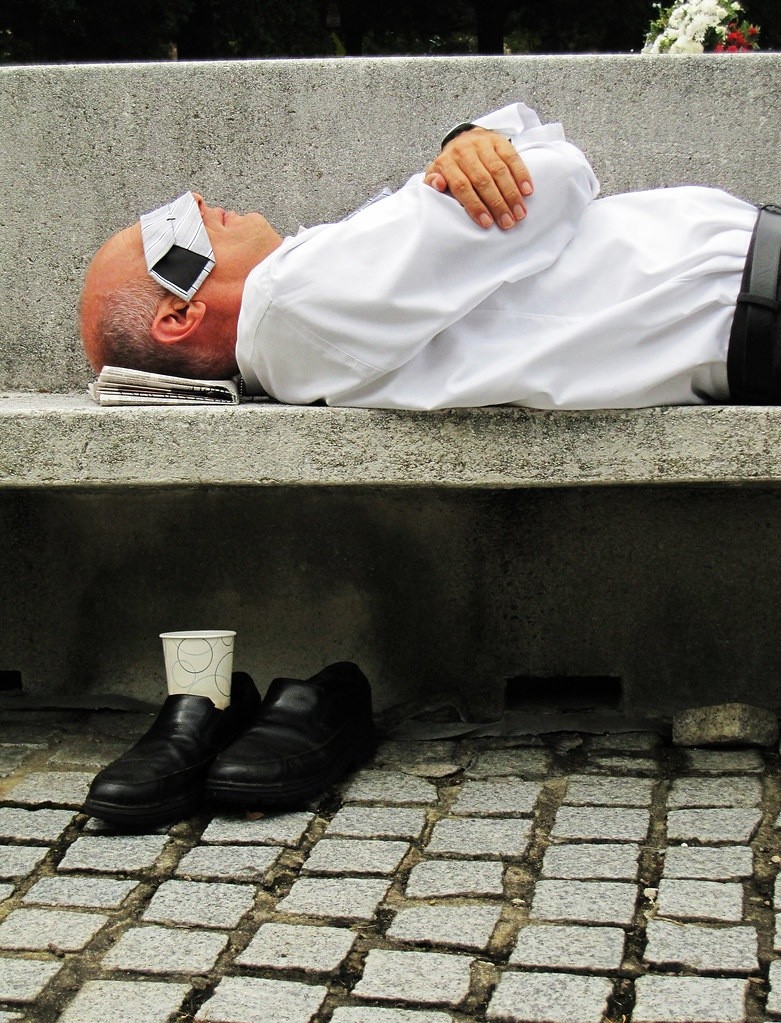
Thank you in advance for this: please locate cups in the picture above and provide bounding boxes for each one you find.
[160,631,236,710]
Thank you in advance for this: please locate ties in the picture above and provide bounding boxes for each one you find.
[140,188,396,304]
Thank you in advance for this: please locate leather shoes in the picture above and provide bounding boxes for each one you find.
[84,673,261,823]
[207,661,373,802]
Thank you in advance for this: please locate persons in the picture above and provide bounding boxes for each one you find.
[79,102,781,413]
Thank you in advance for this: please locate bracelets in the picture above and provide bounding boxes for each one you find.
[441,123,476,151]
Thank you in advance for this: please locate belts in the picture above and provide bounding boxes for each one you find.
[743,208,781,400]
